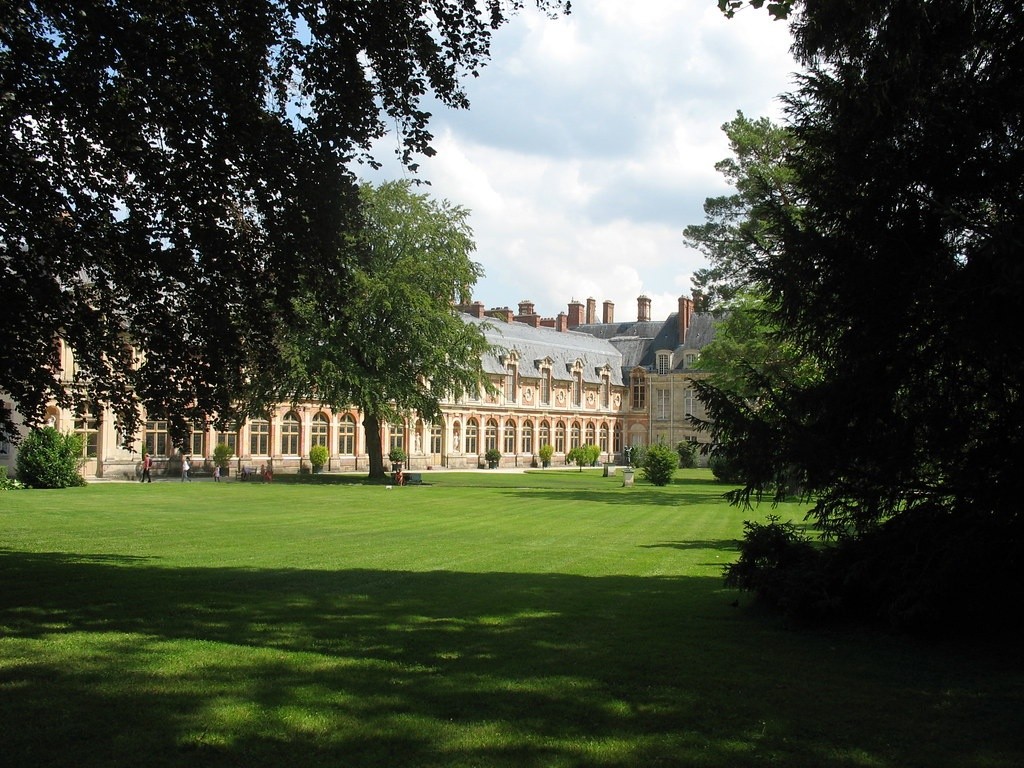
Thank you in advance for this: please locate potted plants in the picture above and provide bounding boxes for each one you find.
[389,447,407,472]
[309,444,329,474]
[213,441,233,476]
[539,444,554,467]
[484,448,502,469]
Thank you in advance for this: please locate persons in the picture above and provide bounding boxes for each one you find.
[212,464,221,482]
[266,460,272,481]
[186,457,191,477]
[241,465,247,481]
[395,468,403,486]
[181,456,191,483]
[261,464,265,482]
[141,453,152,483]
[624,446,634,466]
